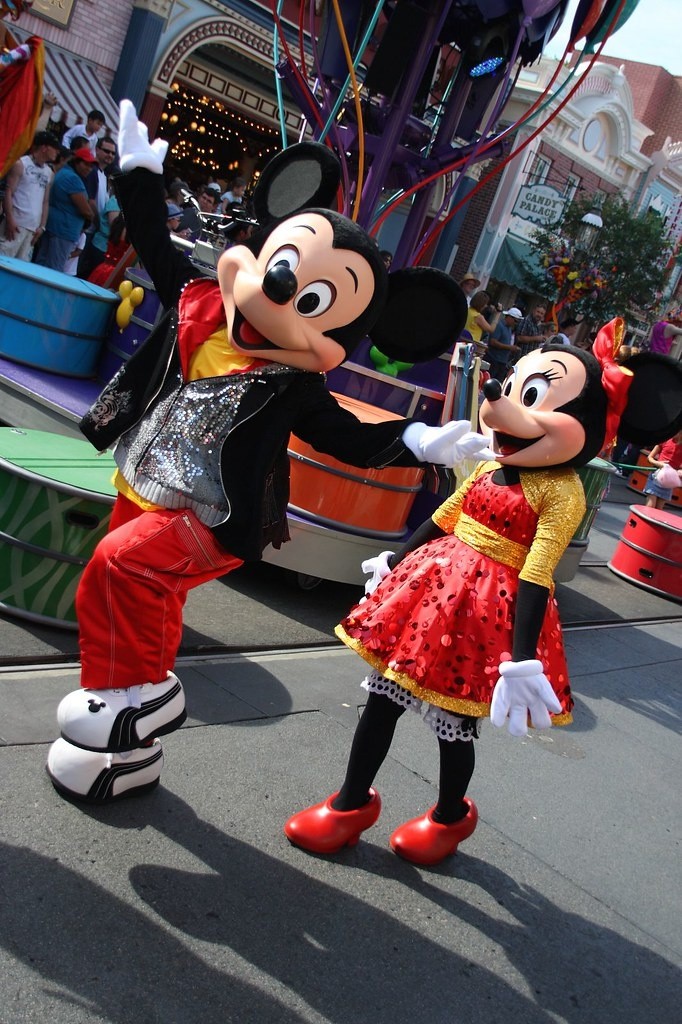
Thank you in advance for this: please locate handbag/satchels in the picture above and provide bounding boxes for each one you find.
[652,464,682,488]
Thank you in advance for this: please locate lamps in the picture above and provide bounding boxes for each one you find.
[574,194,610,253]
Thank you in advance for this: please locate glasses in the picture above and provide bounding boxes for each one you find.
[100,148,116,156]
[466,283,475,288]
[549,329,556,333]
[175,217,181,223]
[511,317,518,323]
[384,258,392,263]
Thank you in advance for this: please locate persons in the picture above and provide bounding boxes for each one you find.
[0,102,681,510]
[47,99,497,807]
[285,317,682,867]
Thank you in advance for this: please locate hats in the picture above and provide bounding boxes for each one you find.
[74,147,100,169]
[542,325,555,335]
[208,182,221,193]
[34,131,66,150]
[503,308,524,319]
[458,274,480,288]
[168,204,184,220]
[170,181,193,195]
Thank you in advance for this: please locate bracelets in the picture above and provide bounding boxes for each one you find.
[504,345,506,350]
[492,320,498,324]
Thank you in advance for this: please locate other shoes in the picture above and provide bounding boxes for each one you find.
[618,467,627,478]
